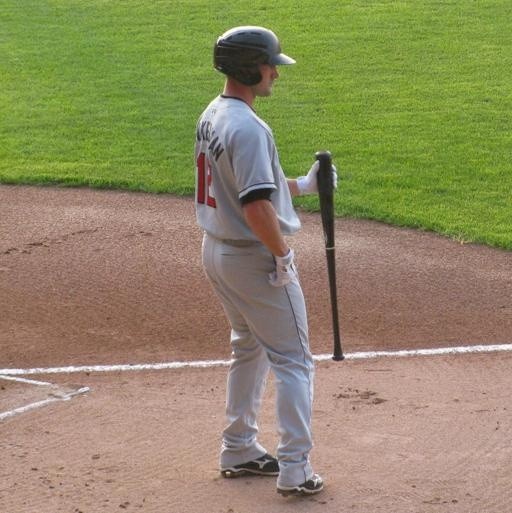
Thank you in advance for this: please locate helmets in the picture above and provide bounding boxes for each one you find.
[213,26,296,86]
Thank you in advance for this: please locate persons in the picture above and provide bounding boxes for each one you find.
[194,25,338,498]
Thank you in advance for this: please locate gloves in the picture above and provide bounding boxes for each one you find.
[267,248,297,288]
[296,159,338,197]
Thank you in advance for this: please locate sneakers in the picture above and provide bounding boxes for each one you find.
[220,453,279,478]
[276,473,323,497]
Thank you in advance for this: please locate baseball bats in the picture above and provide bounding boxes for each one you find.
[315,152,345,361]
